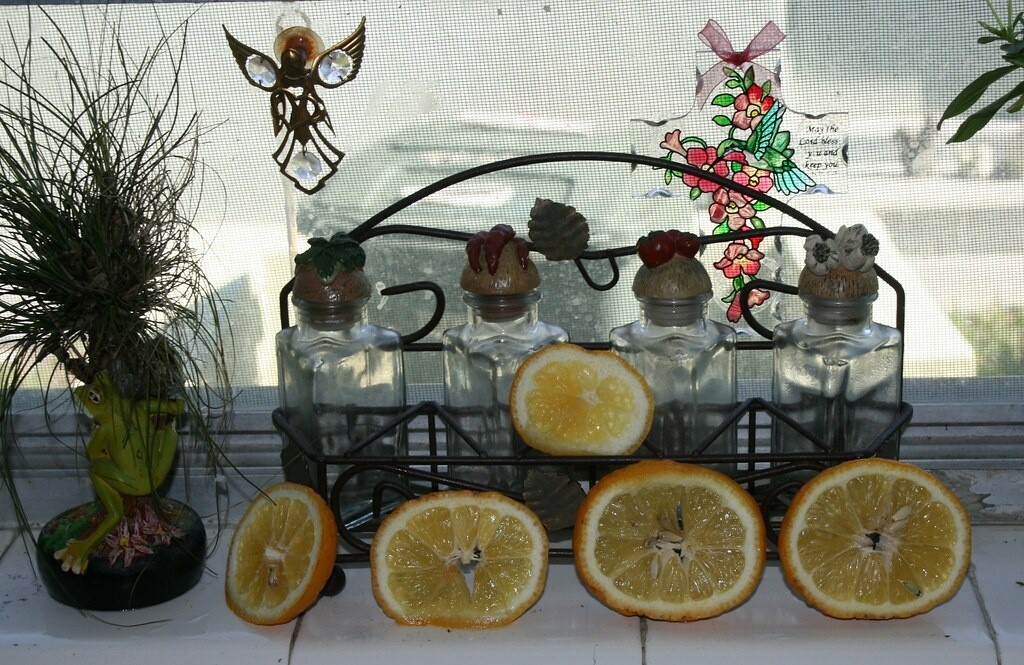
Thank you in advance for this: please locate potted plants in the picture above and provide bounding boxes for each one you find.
[0,0,237,615]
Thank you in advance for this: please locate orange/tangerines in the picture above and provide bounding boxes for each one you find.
[370,490,550,629]
[225,482,338,626]
[778,458,973,620]
[509,343,654,456]
[571,461,766,623]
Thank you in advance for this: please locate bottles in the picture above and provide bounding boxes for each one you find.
[609,255,742,476]
[274,254,411,530]
[769,263,905,516]
[441,244,569,494]
